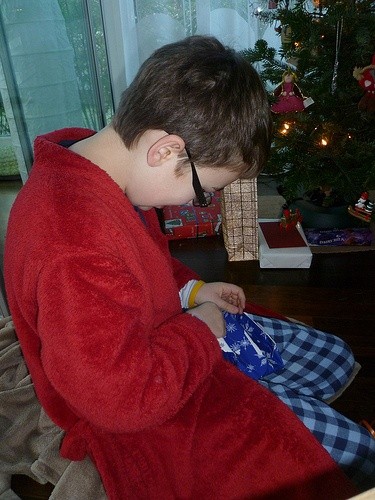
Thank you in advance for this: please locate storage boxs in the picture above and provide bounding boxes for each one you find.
[221,174,312,268]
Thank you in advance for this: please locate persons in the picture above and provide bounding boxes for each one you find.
[2,35,375,500]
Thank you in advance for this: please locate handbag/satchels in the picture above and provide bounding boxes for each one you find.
[220,177,258,261]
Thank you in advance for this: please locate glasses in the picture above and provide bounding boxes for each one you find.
[184,147,212,207]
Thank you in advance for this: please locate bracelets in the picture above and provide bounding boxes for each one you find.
[182,279,205,309]
